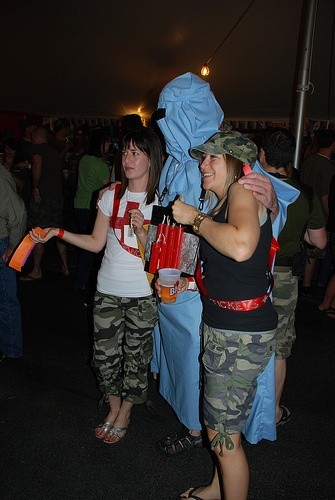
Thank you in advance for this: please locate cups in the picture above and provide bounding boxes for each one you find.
[158,267,181,304]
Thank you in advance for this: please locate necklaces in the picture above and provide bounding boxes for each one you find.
[126,187,146,238]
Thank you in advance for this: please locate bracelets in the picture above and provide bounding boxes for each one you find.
[192,212,213,235]
[32,183,38,188]
[56,228,64,239]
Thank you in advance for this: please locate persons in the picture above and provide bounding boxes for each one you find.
[0,163,28,362]
[95,72,301,456]
[0,114,335,321]
[29,129,164,444]
[172,131,279,500]
[256,127,328,430]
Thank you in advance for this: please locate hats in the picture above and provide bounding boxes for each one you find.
[189,129,259,170]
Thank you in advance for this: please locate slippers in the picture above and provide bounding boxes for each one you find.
[93,410,122,440]
[178,484,231,499]
[103,414,130,443]
